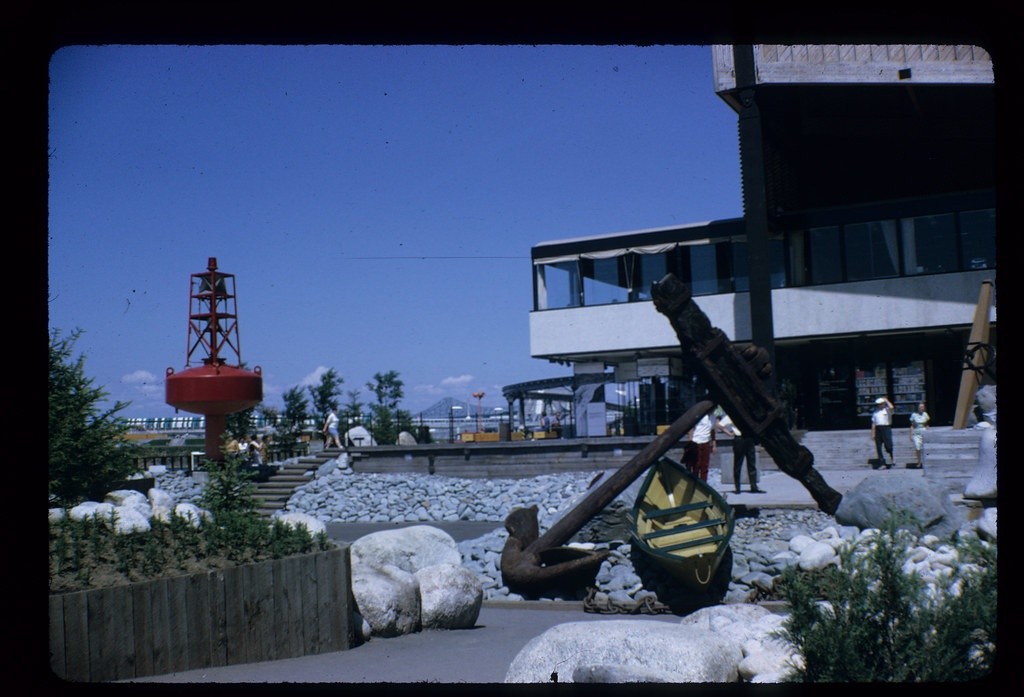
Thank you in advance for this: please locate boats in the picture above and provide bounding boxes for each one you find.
[630,454,738,592]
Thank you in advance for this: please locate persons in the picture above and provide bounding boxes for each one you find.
[550,412,565,440]
[871,396,894,469]
[719,414,767,494]
[909,403,930,468]
[686,414,717,482]
[226,435,270,468]
[530,411,550,441]
[323,407,345,450]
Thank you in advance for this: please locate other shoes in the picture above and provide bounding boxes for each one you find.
[891,457,896,464]
[878,465,887,470]
[751,488,766,493]
[736,489,740,494]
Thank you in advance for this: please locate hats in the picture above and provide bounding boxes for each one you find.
[875,398,884,404]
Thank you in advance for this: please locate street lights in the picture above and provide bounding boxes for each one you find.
[472,391,486,432]
